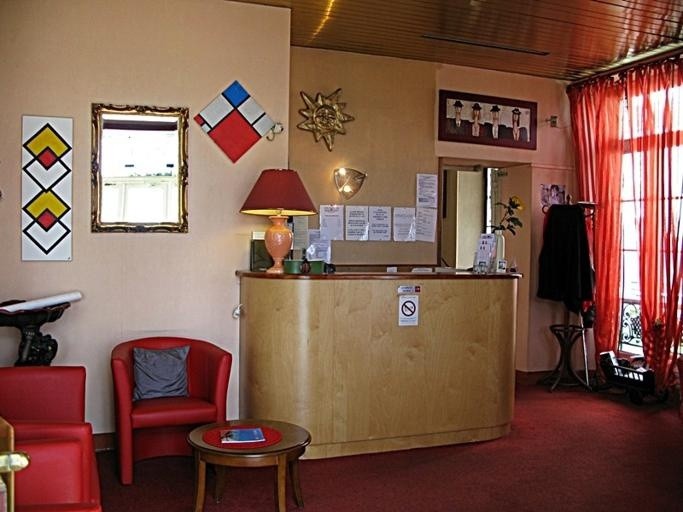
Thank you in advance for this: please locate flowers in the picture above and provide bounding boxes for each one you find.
[490,194,526,236]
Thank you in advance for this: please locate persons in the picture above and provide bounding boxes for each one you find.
[543,185,562,206]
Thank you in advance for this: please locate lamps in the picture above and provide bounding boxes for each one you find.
[334,167,365,200]
[238,169,319,273]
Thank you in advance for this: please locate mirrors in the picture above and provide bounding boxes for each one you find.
[89,102,188,233]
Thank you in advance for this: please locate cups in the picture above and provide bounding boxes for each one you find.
[284,260,336,275]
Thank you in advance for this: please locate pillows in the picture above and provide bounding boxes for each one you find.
[132,342,190,403]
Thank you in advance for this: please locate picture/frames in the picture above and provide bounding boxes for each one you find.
[438,88,538,151]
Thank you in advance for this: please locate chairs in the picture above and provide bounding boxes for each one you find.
[109,336,233,484]
[0,364,102,512]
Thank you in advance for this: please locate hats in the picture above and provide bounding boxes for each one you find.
[490,106,500,112]
[473,104,482,110]
[454,101,463,107]
[513,108,521,115]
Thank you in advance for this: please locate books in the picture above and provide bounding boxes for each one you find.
[475,233,496,274]
[218,427,265,443]
